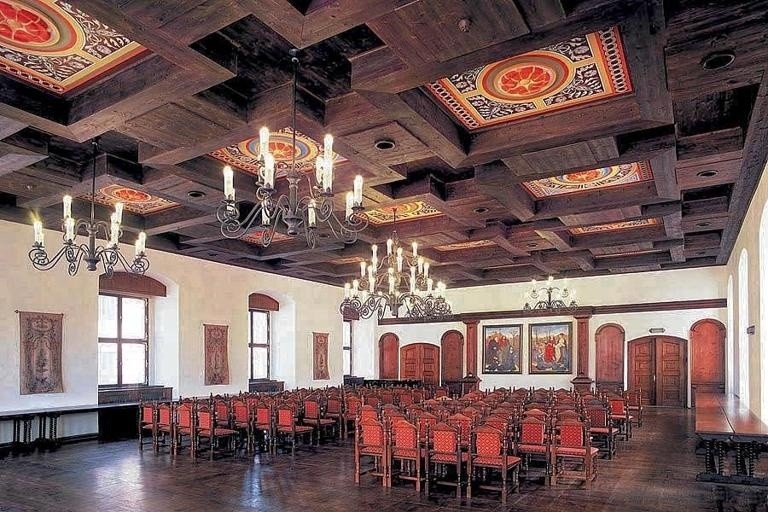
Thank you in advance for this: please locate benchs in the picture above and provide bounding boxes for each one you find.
[688,386,735,487]
[710,389,768,491]
[0,396,186,460]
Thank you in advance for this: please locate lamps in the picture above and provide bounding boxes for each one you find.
[333,204,458,325]
[210,46,374,251]
[516,273,582,317]
[24,131,156,282]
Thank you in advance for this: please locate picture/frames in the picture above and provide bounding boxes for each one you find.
[527,320,573,376]
[479,322,524,374]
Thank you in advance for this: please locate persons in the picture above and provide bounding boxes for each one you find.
[536,333,568,371]
[487,333,516,371]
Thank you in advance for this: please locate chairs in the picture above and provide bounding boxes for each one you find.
[353,383,644,506]
[139,384,354,470]
